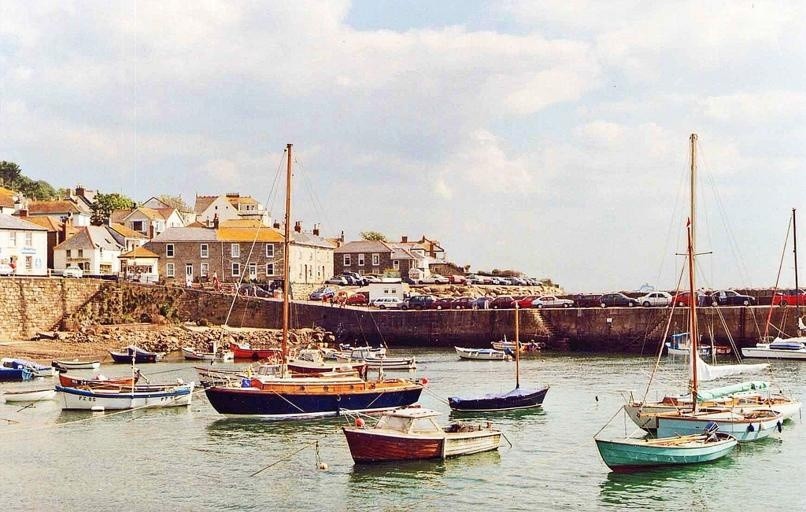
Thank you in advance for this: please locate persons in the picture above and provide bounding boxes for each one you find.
[252,284,257,297]
[186,279,192,289]
[206,270,218,284]
[8,257,16,278]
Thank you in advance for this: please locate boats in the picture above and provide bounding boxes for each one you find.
[449,386,549,412]
[341,407,502,463]
[182,342,273,359]
[0,359,195,410]
[455,340,545,359]
[106,346,165,363]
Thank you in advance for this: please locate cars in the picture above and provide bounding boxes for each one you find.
[595,288,806,308]
[63,265,83,278]
[311,271,574,308]
